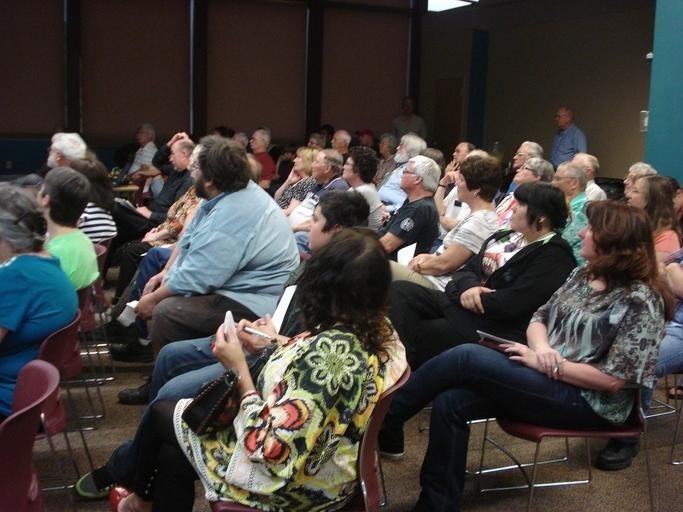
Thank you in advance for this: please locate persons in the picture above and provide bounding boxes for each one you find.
[379,201,675,509]
[112,228,404,511]
[549,107,584,169]
[0,125,682,468]
[72,192,368,502]
[389,95,425,142]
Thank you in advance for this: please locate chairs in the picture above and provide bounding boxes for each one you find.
[0,242,104,512]
[207,366,410,512]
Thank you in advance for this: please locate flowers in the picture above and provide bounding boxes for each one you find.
[104,167,132,187]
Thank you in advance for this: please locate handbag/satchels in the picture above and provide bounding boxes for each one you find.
[182,346,274,438]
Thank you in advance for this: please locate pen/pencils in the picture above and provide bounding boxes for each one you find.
[554,343,566,374]
[244,327,271,339]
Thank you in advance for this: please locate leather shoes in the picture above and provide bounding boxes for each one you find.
[78,306,156,511]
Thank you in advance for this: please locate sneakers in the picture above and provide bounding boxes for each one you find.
[597,438,640,470]
[378,431,405,460]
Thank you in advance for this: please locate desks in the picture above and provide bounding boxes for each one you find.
[111,184,139,207]
